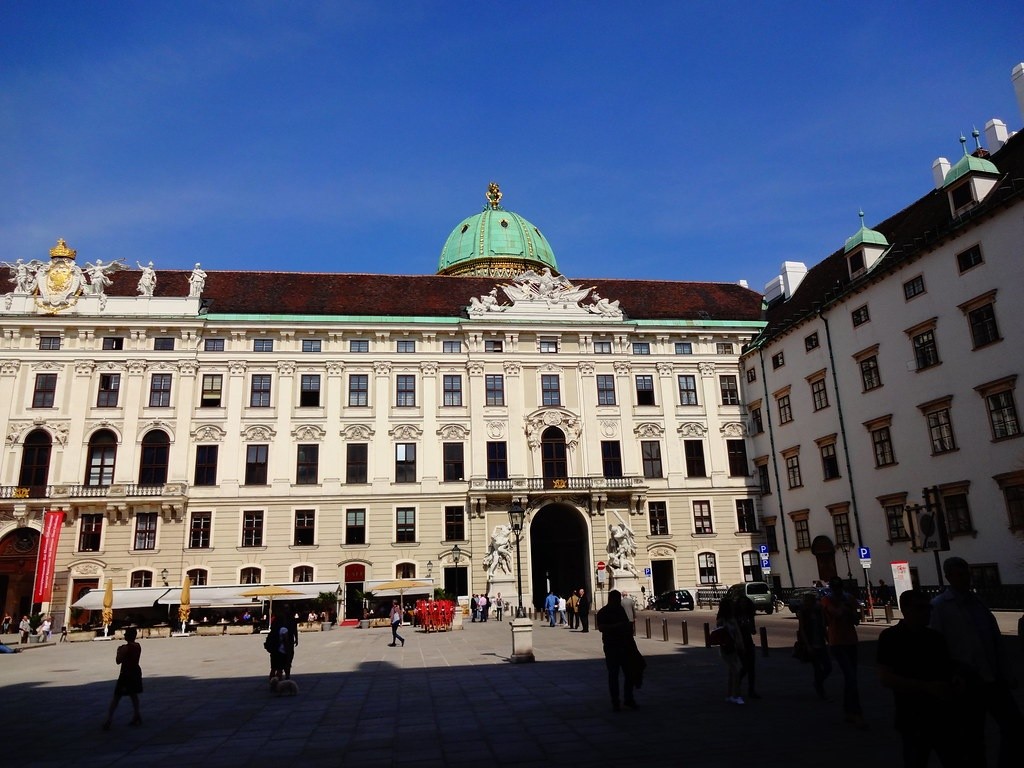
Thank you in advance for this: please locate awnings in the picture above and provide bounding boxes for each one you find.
[71,579,434,610]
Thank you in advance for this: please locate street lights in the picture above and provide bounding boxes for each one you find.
[451,545,461,606]
[427,561,433,577]
[507,501,526,617]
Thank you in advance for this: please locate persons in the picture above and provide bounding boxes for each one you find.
[529,420,544,447]
[717,577,871,729]
[0,613,51,654]
[545,589,590,633]
[243,611,318,621]
[4,259,207,297]
[610,526,641,575]
[596,590,647,713]
[565,420,578,449]
[263,604,298,695]
[471,592,504,622]
[489,524,513,576]
[877,580,894,618]
[388,600,404,647]
[102,627,143,731]
[877,557,1024,768]
[356,602,418,629]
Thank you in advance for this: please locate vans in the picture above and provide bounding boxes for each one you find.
[726,582,773,615]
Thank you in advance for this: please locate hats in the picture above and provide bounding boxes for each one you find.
[943,557,968,572]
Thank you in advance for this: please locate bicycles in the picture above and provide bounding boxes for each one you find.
[773,595,785,611]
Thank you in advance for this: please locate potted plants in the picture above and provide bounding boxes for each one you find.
[354,589,377,630]
[314,592,337,630]
[26,611,46,644]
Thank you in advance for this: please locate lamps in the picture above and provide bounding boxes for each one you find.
[427,560,433,577]
[161,569,169,586]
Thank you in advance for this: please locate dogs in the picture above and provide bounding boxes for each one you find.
[269,677,298,696]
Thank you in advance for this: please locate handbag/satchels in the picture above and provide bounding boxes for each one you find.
[487,601,491,607]
[264,636,281,653]
[791,629,810,662]
[567,605,574,613]
[392,612,400,625]
[708,625,731,646]
[478,606,483,611]
[48,630,51,639]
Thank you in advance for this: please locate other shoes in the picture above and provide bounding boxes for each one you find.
[550,621,589,632]
[726,696,736,702]
[733,697,745,705]
[472,618,487,622]
[852,716,867,728]
[388,643,396,647]
[624,699,639,710]
[822,695,835,704]
[612,706,622,712]
[835,696,848,710]
[812,681,818,690]
[402,639,404,647]
[749,692,761,700]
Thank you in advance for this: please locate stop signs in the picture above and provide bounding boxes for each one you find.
[597,562,606,570]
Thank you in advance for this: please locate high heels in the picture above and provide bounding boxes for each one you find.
[129,716,143,726]
[102,721,111,730]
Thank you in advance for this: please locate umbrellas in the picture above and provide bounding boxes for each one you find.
[370,577,429,622]
[102,578,113,637]
[179,575,191,634]
[239,584,306,630]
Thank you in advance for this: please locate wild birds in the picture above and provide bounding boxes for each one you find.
[513,267,572,294]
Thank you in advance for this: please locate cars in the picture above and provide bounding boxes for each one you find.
[789,587,865,623]
[654,589,694,611]
